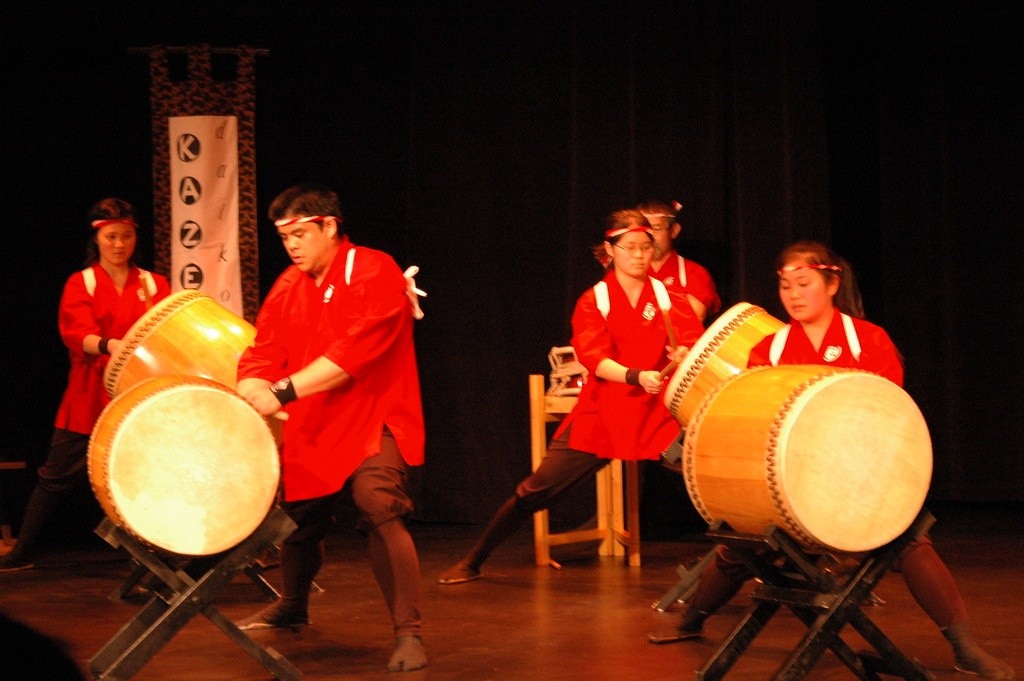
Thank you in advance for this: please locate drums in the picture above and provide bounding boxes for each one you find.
[681,363,935,554]
[86,372,281,558]
[103,288,281,450]
[664,302,790,434]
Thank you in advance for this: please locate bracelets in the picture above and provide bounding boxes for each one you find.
[268,376,297,407]
[626,368,641,386]
[98,336,111,354]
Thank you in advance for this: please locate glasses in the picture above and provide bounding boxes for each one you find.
[648,224,672,236]
[611,242,656,255]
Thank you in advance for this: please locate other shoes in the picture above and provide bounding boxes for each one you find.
[248,546,282,568]
[648,627,708,643]
[942,650,1015,681]
[551,548,599,569]
[0,555,35,572]
[130,558,151,593]
[438,567,484,584]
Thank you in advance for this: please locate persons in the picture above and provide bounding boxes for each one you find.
[236,185,427,671]
[635,201,720,324]
[0,197,172,573]
[437,209,706,584]
[649,241,1016,681]
